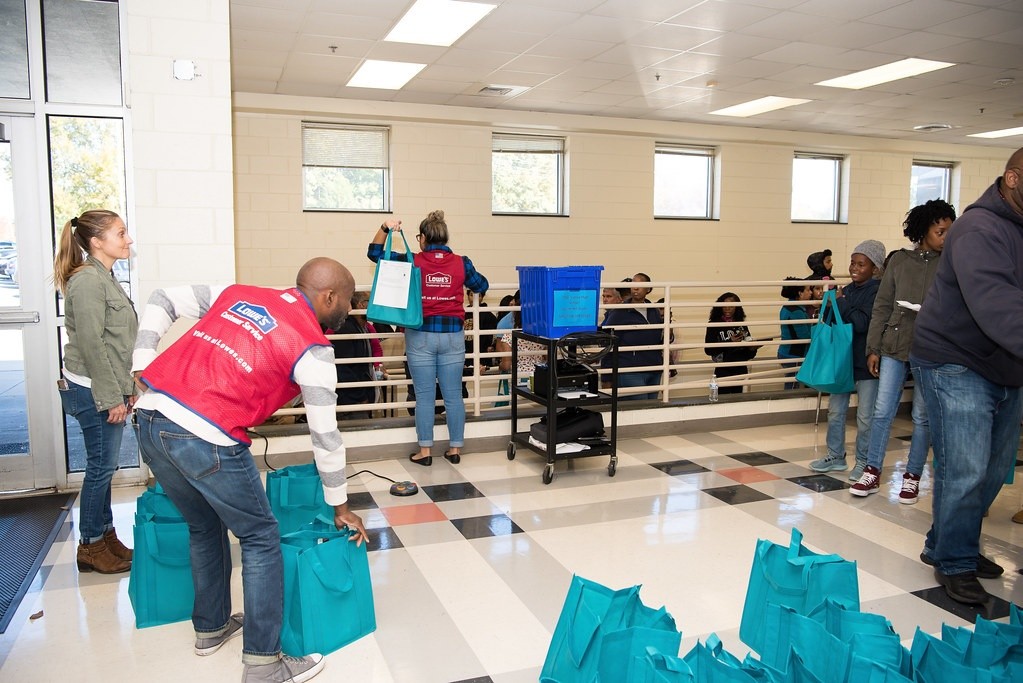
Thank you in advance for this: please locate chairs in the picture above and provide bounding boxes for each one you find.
[378,337,408,418]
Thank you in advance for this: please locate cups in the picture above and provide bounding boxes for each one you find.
[530,371,535,392]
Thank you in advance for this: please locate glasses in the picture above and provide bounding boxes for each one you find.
[416,234,422,242]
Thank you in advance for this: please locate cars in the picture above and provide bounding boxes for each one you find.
[0,243,131,294]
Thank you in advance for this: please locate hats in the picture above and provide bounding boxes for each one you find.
[852,239,886,270]
[616,278,632,291]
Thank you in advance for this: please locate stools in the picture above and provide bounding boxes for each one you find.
[494,374,529,406]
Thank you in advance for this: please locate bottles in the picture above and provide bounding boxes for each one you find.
[375,364,386,381]
[709,374,719,403]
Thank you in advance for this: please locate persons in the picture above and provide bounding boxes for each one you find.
[804,249,838,295]
[463,290,498,376]
[130,257,370,683]
[907,145,1022,607]
[704,292,756,395]
[600,286,623,326]
[499,295,515,321]
[368,210,489,466]
[777,276,812,390]
[500,299,547,376]
[807,277,823,318]
[847,197,956,504]
[657,298,677,378]
[496,290,520,352]
[367,323,388,377]
[809,240,888,481]
[334,291,373,419]
[604,273,664,401]
[56,210,139,575]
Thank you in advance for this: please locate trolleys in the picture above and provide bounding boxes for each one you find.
[507,329,621,484]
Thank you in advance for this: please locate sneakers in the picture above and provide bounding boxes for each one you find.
[849,465,881,496]
[899,472,920,503]
[194,612,244,655]
[809,450,848,472]
[242,653,325,683]
[849,463,866,480]
[921,551,1004,578]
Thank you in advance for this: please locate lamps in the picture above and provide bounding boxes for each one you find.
[172,58,196,80]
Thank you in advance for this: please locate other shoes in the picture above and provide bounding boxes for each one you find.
[445,451,460,464]
[410,453,432,466]
[104,527,133,561]
[77,539,132,574]
[1012,510,1023,523]
[934,572,986,603]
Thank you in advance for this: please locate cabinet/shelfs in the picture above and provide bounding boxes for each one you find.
[507,328,621,485]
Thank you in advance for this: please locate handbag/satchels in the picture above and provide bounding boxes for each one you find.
[366,229,424,329]
[280,513,376,655]
[540,528,1023,683]
[789,344,806,357]
[795,289,855,393]
[266,463,335,535]
[495,378,509,407]
[128,480,195,629]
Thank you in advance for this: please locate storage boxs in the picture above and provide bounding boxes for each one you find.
[529,375,534,394]
[516,265,606,339]
[534,360,599,399]
